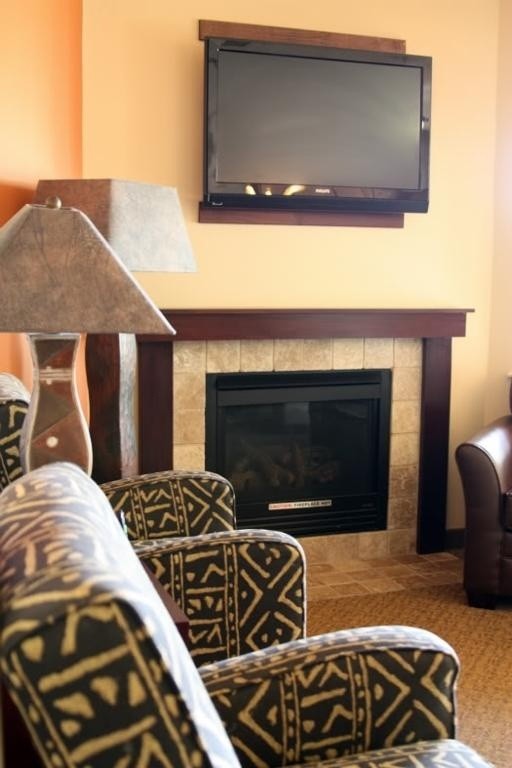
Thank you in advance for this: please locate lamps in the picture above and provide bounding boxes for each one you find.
[34,179,199,271]
[0,195,177,478]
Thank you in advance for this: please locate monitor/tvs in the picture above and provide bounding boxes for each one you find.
[203,36,432,214]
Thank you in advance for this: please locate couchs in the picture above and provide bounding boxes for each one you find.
[0,371,306,669]
[1,463,494,768]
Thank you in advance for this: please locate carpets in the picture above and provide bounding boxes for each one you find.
[305,583,511,768]
